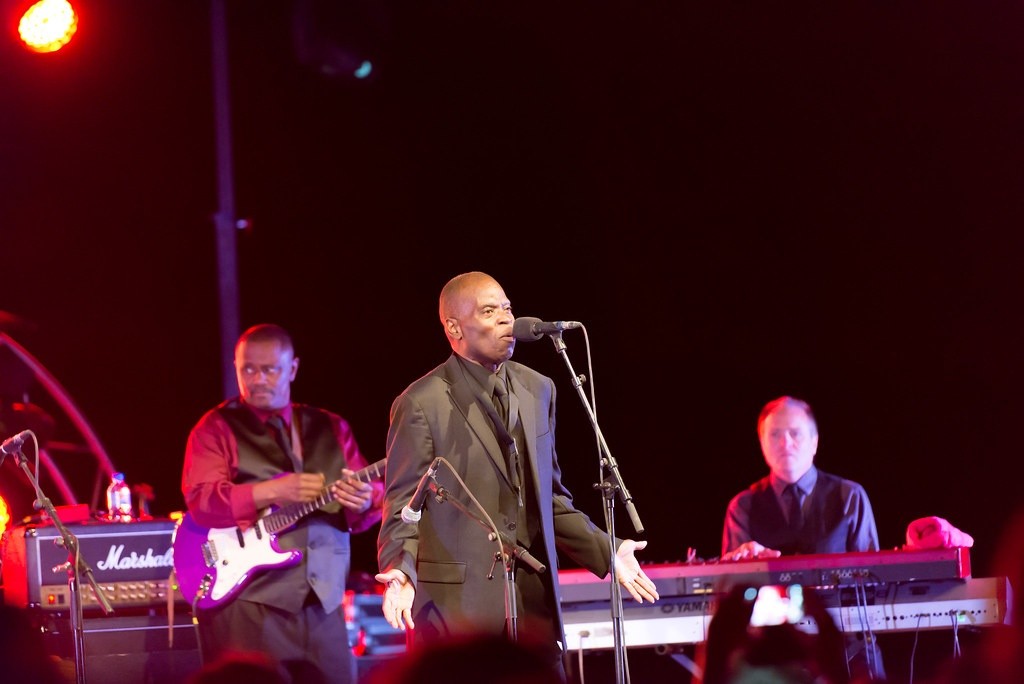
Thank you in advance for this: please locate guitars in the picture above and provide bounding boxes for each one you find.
[174,457,388,611]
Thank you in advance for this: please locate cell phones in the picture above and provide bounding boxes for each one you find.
[732,580,805,627]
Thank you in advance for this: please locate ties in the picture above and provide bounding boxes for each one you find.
[786,484,804,529]
[264,415,299,474]
[494,375,511,426]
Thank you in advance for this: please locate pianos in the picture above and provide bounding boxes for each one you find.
[555,546,1018,654]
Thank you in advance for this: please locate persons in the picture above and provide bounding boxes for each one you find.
[721,394,880,564]
[376,272,660,684]
[181,324,386,683]
[0,312,56,525]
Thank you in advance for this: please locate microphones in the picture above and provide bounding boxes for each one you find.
[512,317,582,341]
[0,430,30,466]
[400,458,441,523]
